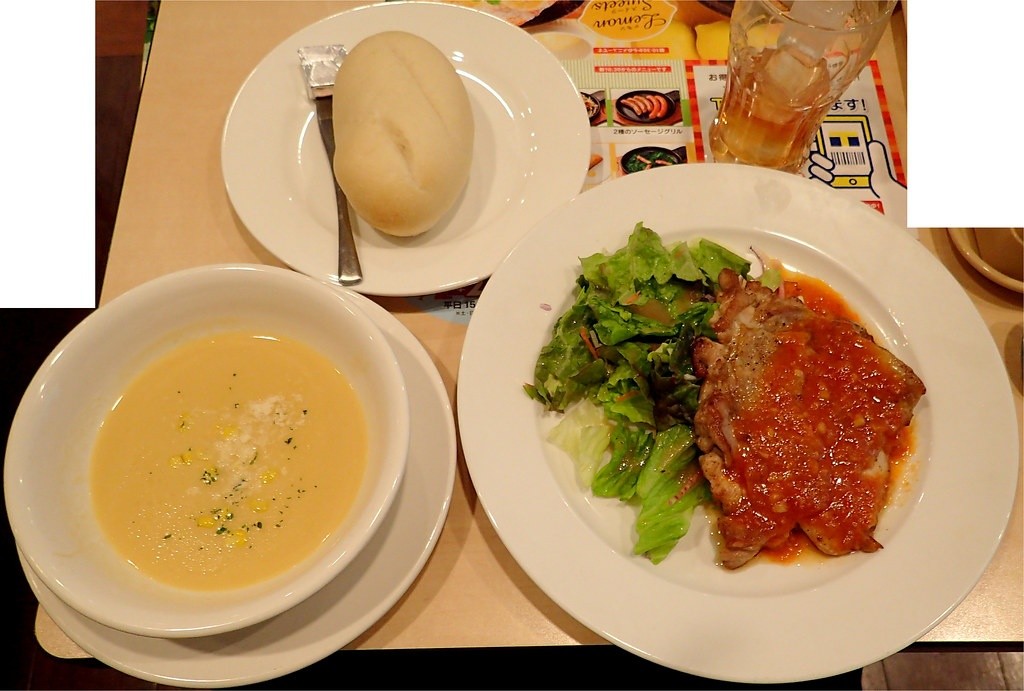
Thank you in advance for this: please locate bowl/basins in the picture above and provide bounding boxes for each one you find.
[3,263,412,637]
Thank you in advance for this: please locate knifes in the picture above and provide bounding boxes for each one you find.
[316,98,363,283]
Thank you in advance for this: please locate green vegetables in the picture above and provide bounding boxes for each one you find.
[626,151,674,172]
[525,215,779,568]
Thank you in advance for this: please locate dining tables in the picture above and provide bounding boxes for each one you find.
[36,0,1024,659]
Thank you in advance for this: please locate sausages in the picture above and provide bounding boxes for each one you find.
[620,95,667,119]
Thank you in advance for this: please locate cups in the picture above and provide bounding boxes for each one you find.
[708,1,896,174]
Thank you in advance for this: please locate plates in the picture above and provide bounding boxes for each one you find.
[456,163,1020,683]
[16,288,455,689]
[946,228,1024,295]
[220,2,591,297]
